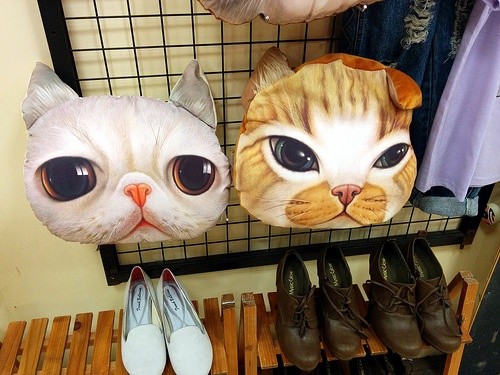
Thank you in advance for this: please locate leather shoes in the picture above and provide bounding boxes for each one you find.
[368,239,421,358]
[276,250,320,370]
[316,244,361,361]
[403,237,462,353]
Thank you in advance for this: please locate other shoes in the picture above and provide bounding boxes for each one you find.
[156,268,212,374]
[120,266,166,374]
